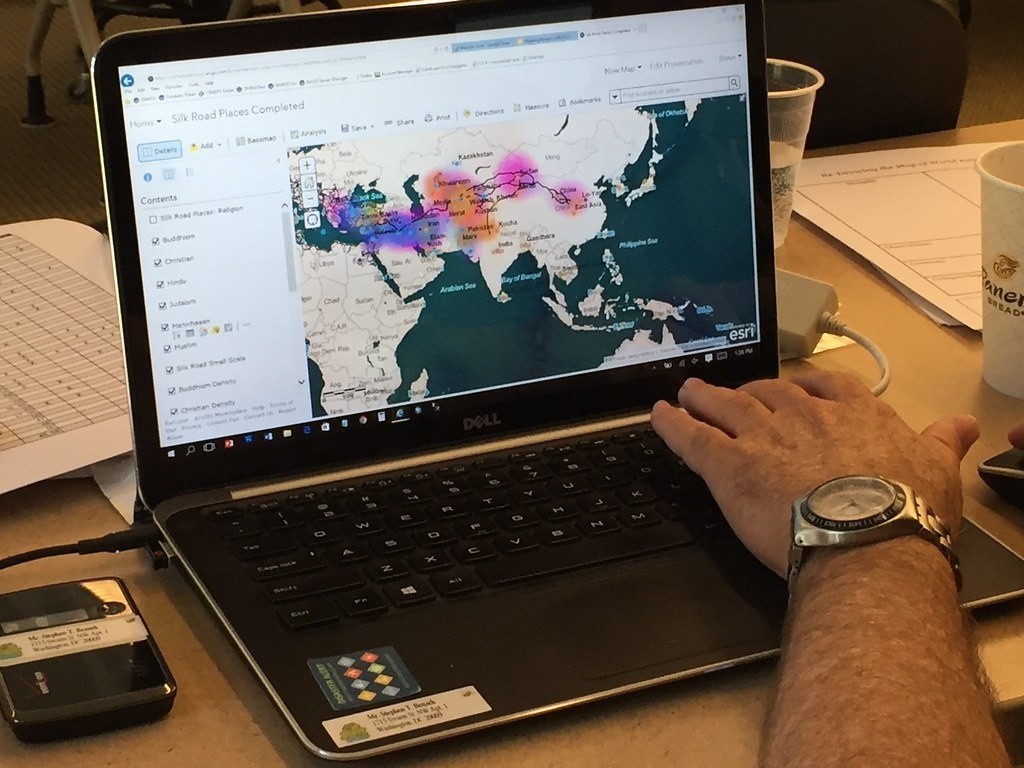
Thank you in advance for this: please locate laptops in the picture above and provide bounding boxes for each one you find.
[90,0,1024,760]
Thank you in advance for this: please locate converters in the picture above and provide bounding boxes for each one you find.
[776,268,837,359]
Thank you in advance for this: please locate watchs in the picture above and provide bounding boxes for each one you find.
[786,474,963,607]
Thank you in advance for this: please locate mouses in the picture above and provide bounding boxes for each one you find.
[976,445,1024,509]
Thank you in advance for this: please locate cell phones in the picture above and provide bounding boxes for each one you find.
[0,576,178,741]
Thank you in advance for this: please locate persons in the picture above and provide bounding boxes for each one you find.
[650,369,1024,768]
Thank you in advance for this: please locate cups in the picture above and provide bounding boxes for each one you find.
[766,58,825,248]
[975,141,1024,400]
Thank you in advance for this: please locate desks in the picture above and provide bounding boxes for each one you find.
[1,121,1024,768]
